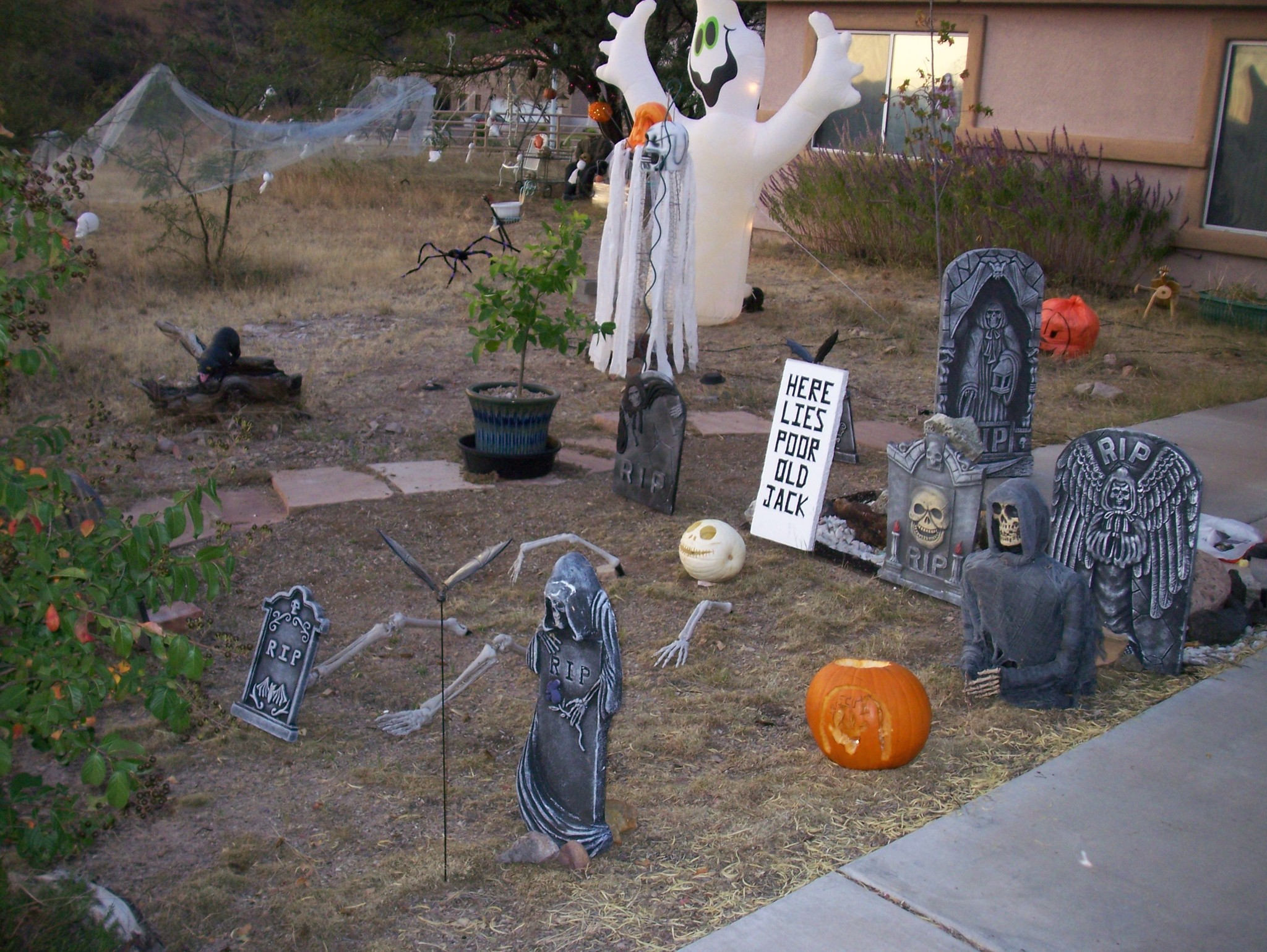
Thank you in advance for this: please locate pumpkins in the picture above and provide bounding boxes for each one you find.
[595,175,603,182]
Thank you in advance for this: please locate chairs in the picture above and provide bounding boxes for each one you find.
[482,195,520,256]
[498,134,546,190]
[563,139,609,200]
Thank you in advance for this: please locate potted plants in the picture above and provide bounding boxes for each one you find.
[457,211,617,479]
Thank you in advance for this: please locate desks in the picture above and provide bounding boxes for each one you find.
[592,182,630,210]
